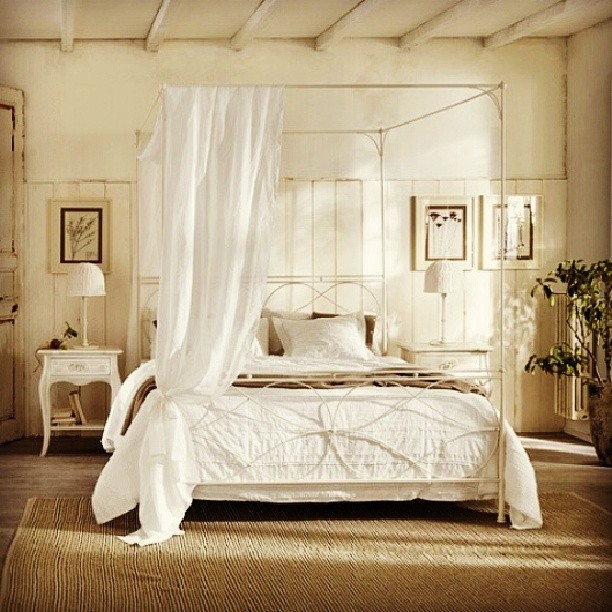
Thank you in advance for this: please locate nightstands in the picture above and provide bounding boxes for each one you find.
[36,344,124,458]
[398,344,495,399]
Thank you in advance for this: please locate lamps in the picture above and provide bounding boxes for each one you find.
[423,259,461,346]
[63,260,105,350]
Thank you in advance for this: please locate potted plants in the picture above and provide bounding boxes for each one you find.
[524,260,611,467]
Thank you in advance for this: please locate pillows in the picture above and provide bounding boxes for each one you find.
[271,312,375,363]
[254,317,270,354]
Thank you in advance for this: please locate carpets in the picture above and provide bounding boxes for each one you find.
[0,497,612,611]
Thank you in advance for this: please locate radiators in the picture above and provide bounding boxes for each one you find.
[550,282,591,421]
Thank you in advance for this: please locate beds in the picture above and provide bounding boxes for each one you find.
[90,81,544,547]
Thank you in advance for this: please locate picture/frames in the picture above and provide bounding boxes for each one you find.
[411,194,474,272]
[478,193,546,271]
[46,197,113,274]
